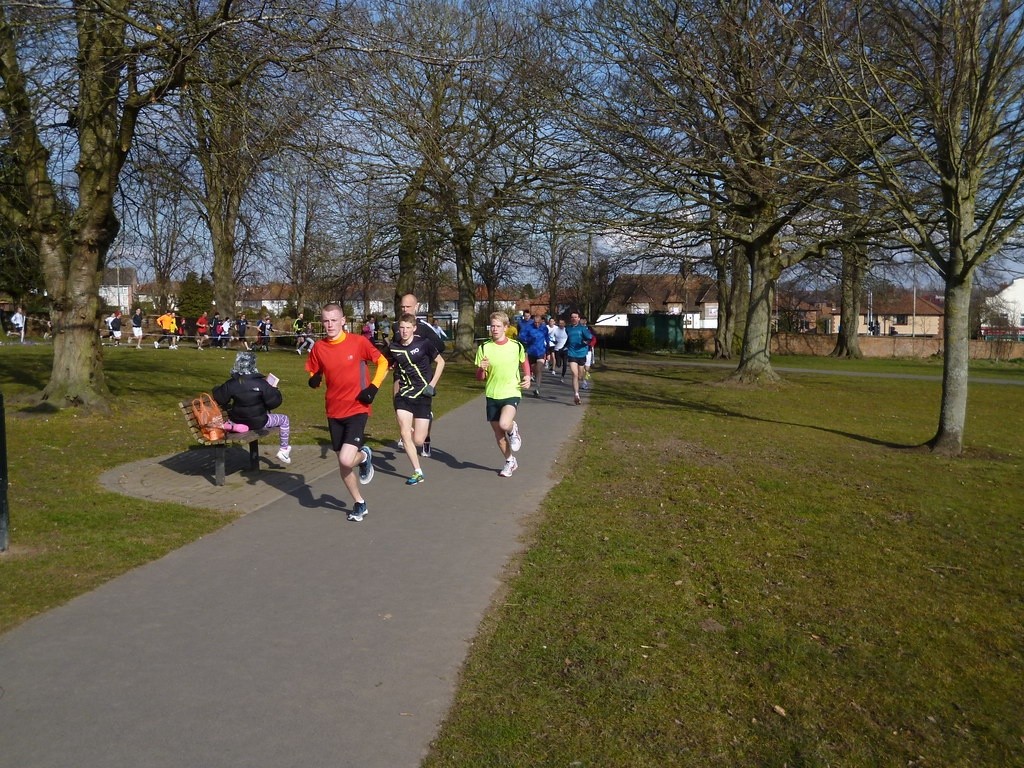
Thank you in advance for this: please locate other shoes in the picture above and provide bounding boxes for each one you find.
[108,335,269,350]
[533,390,541,397]
[293,348,312,356]
[7,330,13,337]
[530,376,535,381]
[560,379,565,383]
[574,393,580,404]
[552,370,555,375]
[585,372,590,380]
[545,359,552,370]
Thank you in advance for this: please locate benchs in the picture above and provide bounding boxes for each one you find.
[178,396,280,485]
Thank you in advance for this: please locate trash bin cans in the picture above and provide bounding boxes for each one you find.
[441,330,452,339]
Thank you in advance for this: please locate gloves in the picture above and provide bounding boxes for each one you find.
[355,383,378,405]
[308,370,323,389]
[422,385,434,398]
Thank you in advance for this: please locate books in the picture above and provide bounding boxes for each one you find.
[266,373,280,386]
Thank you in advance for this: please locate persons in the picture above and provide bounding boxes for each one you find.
[100,308,391,355]
[387,294,448,486]
[474,312,530,477]
[7,308,25,342]
[305,303,389,521]
[505,309,597,405]
[212,352,291,464]
[44,322,52,339]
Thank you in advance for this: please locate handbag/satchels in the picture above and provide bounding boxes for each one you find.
[191,393,225,441]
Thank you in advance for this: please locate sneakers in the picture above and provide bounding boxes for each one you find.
[507,421,521,451]
[276,446,292,464]
[421,441,431,457]
[499,457,519,477]
[398,439,405,450]
[580,381,589,390]
[358,446,374,485]
[405,471,424,485]
[347,502,368,522]
